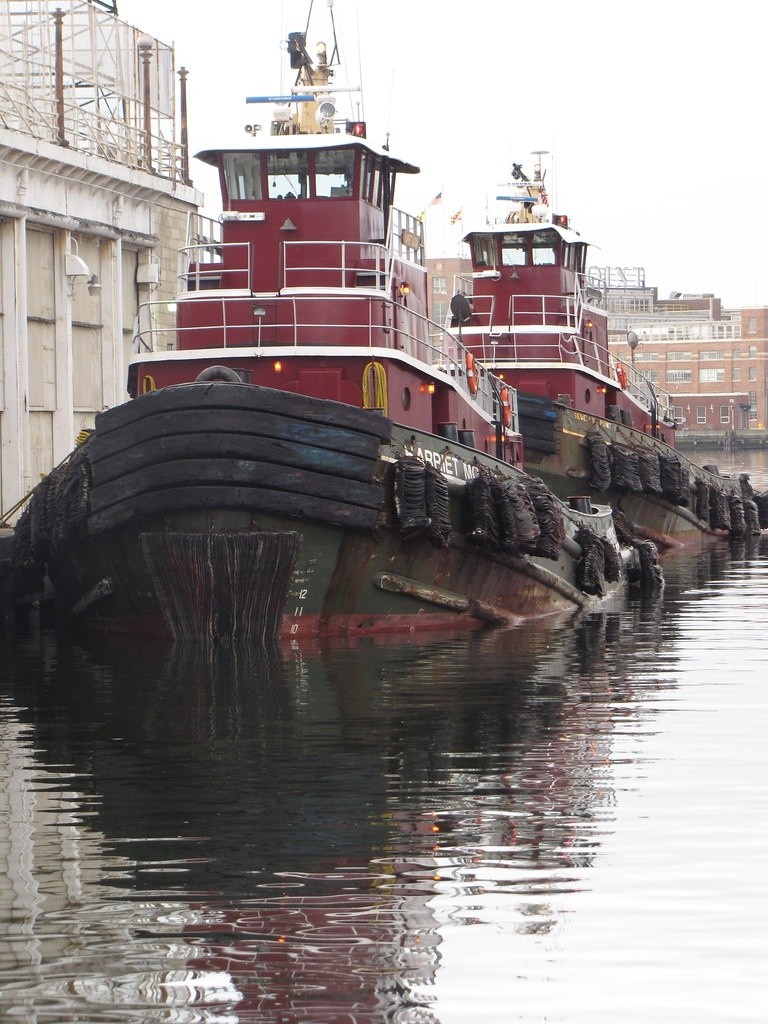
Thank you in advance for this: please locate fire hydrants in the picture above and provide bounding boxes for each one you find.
[758,423,763,431]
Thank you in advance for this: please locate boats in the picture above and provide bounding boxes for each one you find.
[0,0,667,739]
[440,151,768,566]
